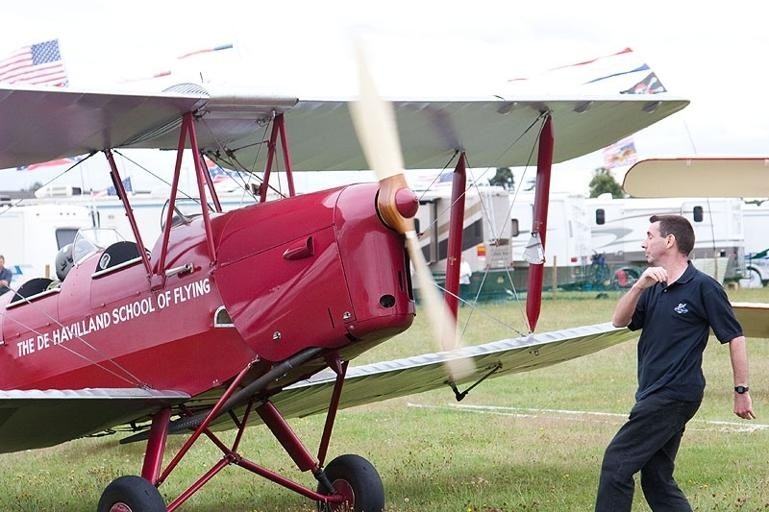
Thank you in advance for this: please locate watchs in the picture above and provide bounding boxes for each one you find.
[735,385,749,394]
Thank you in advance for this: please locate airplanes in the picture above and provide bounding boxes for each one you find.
[0,87,692,511]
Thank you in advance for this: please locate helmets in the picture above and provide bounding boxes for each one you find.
[56,240,94,281]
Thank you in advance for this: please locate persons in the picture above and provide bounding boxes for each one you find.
[0,255,12,297]
[592,213,758,512]
